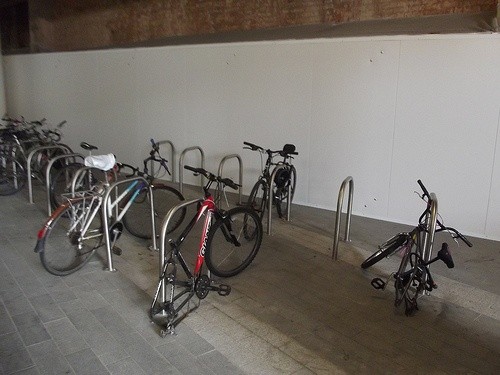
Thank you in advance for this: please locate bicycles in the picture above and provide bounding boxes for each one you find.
[204,141,298,278]
[148,164,263,338]
[33,137,187,276]
[0,112,154,237]
[360,179,473,317]
